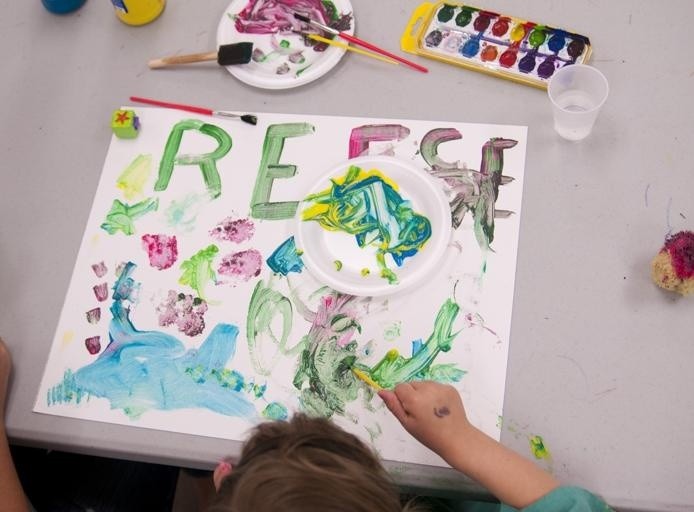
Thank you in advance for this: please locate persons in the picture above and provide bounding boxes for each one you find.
[0,338,36,511]
[213,343,611,512]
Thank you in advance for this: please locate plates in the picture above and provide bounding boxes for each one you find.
[294,152,453,298]
[215,0,355,92]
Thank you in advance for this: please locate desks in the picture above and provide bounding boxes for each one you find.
[6,4,694,509]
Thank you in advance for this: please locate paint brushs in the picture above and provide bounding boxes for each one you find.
[290,28,399,65]
[131,96,257,127]
[293,13,429,74]
[345,360,384,391]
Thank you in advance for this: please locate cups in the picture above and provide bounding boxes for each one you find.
[548,62,609,143]
